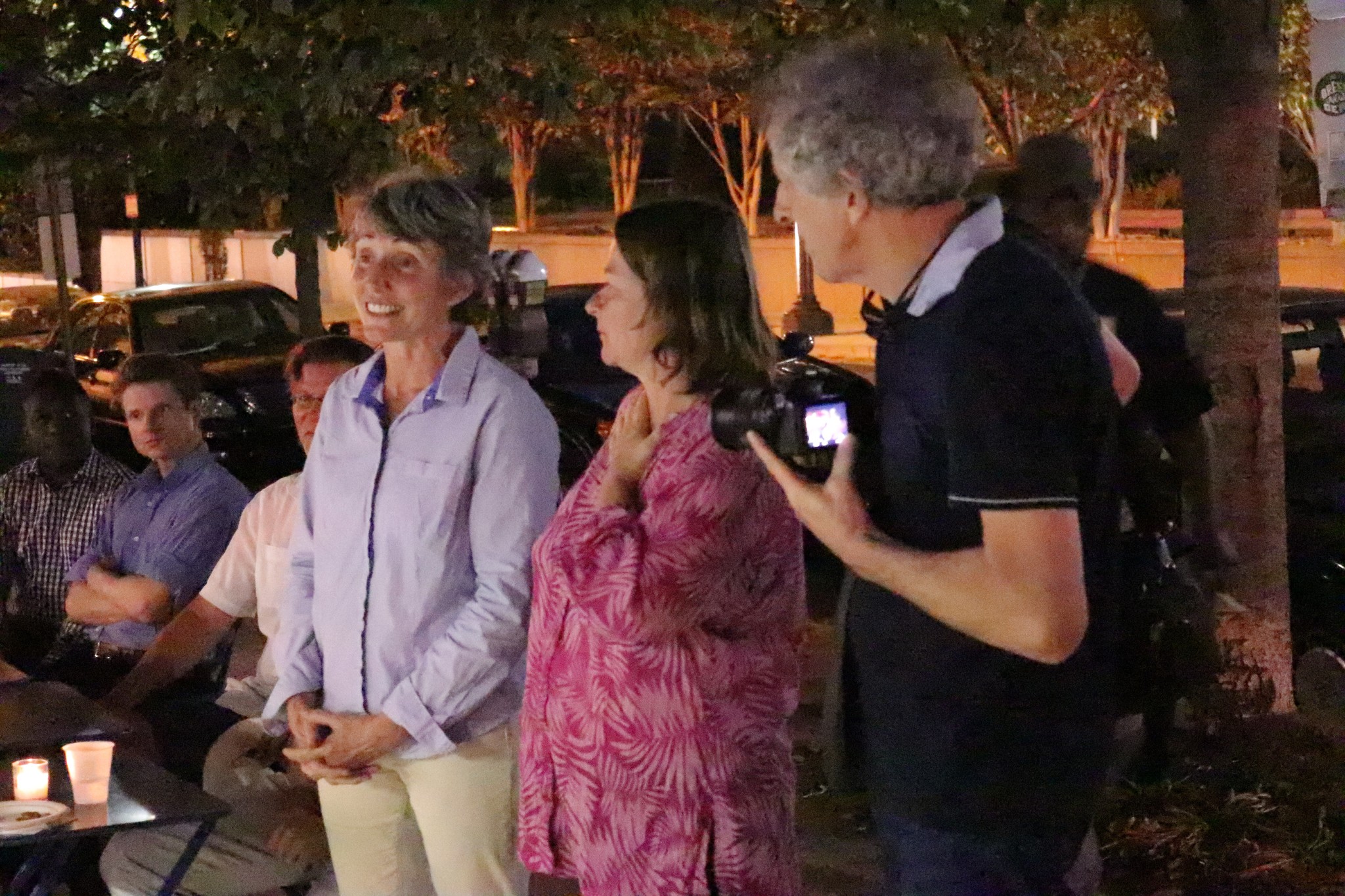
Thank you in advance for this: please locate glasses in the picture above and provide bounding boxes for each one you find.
[289,391,326,410]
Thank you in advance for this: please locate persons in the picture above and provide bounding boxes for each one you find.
[0,332,379,896]
[513,193,808,896]
[277,174,560,896]
[745,27,1217,896]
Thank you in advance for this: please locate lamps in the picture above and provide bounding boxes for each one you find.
[120,185,143,229]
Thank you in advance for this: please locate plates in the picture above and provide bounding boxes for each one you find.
[0,800,67,829]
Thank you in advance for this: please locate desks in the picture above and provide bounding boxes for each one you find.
[0,743,238,896]
[0,678,138,752]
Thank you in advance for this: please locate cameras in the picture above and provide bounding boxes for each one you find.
[711,365,864,482]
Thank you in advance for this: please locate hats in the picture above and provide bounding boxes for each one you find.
[1000,134,1100,202]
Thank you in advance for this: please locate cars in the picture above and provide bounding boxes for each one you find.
[42,278,355,488]
[0,271,105,462]
[1152,281,1345,516]
[474,278,884,561]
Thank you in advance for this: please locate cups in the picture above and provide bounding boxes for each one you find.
[11,759,49,801]
[61,741,116,804]
